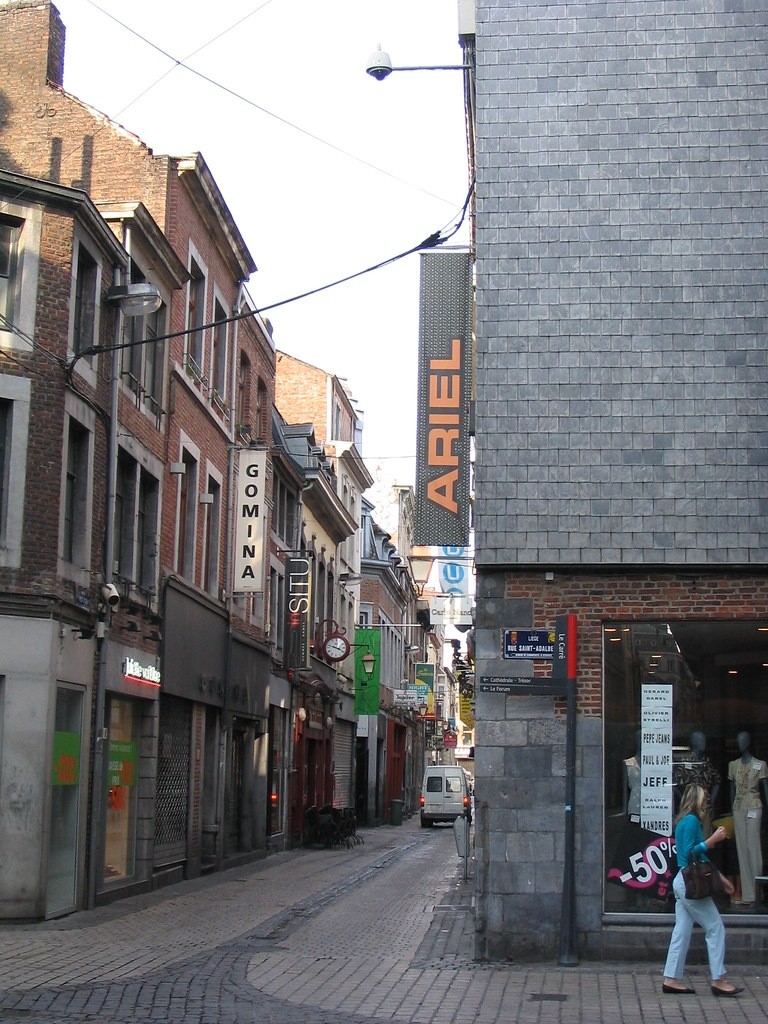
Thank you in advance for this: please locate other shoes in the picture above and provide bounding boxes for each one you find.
[711,986,744,996]
[662,983,695,994]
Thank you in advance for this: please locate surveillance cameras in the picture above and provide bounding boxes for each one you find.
[364,51,392,81]
[102,584,120,607]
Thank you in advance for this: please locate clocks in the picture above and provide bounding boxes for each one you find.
[315,619,350,662]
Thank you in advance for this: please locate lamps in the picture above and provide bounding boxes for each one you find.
[418,697,428,716]
[349,681,368,690]
[407,555,475,597]
[313,692,322,704]
[339,573,362,586]
[350,644,376,681]
[103,283,162,317]
[335,702,343,712]
[437,717,449,730]
[296,708,307,721]
[324,717,333,729]
[404,645,421,658]
[113,572,163,641]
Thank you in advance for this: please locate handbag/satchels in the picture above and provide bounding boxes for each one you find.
[681,847,725,899]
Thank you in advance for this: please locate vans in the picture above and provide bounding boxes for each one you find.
[420,766,473,828]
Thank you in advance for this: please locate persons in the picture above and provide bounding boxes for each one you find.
[727,732,768,905]
[662,784,745,997]
[623,731,651,907]
[672,732,721,853]
